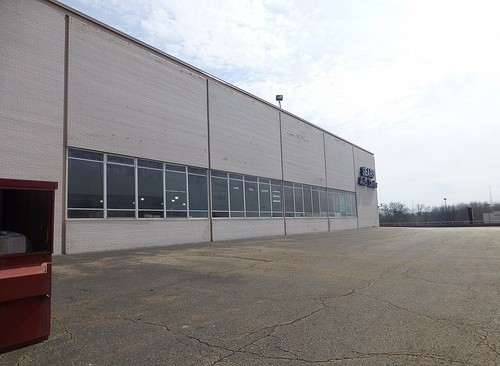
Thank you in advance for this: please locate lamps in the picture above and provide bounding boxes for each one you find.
[275,94,283,108]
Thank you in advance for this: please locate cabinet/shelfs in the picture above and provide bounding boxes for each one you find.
[1,177,58,353]
